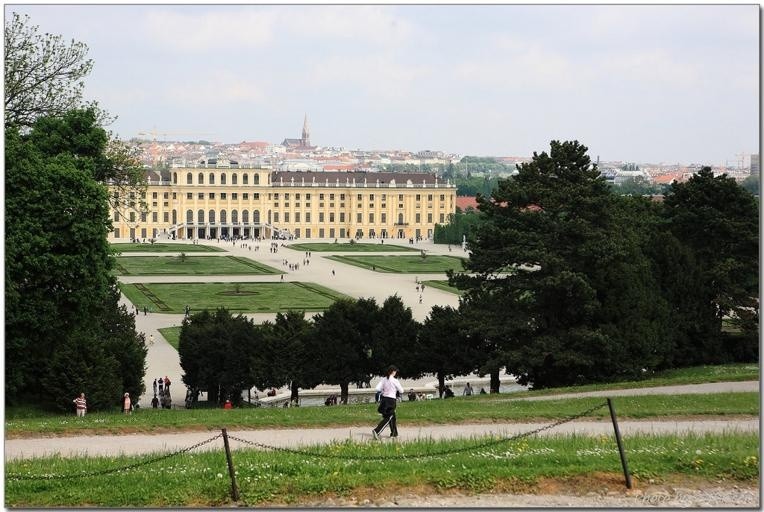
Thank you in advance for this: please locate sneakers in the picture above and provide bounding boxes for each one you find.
[372,429,401,441]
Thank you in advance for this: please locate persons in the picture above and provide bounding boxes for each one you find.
[283,396,300,408]
[415,279,425,304]
[128,234,297,252]
[280,247,313,271]
[335,233,427,246]
[72,392,87,416]
[407,380,472,403]
[324,393,346,407]
[150,376,173,410]
[120,392,134,414]
[371,365,405,440]
